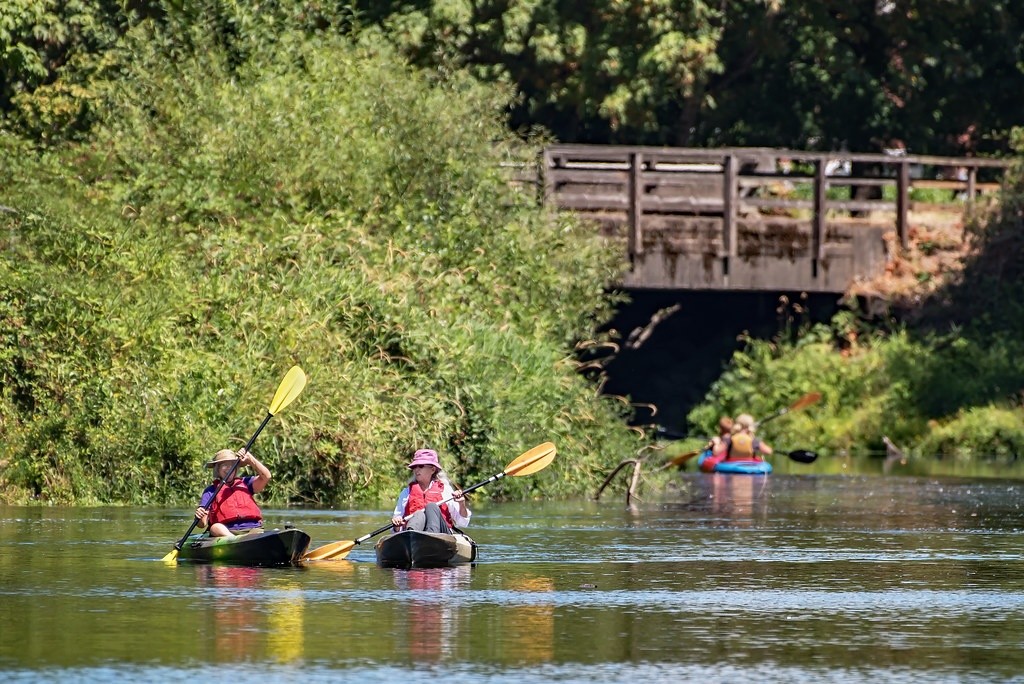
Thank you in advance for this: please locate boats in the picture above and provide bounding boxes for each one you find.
[175,525,310,568]
[698,451,774,475]
[374,527,478,569]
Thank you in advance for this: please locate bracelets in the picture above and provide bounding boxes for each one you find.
[393,527,400,533]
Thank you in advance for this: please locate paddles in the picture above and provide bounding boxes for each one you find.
[162,365,307,563]
[299,442,557,561]
[670,393,820,466]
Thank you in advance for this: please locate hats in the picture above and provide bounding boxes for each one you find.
[408,449,443,471]
[206,449,240,467]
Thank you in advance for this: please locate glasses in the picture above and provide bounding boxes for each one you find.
[219,465,232,470]
[410,465,433,469]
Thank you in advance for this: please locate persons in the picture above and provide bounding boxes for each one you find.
[711,414,773,459]
[194,449,272,537]
[391,449,472,534]
[950,126,981,202]
